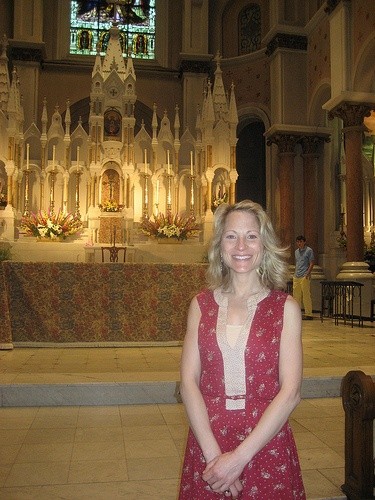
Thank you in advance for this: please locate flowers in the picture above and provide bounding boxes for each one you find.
[139,207,202,243]
[95,196,126,212]
[19,203,84,243]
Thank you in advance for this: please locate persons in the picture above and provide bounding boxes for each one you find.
[291,236,315,320]
[177,200,307,500]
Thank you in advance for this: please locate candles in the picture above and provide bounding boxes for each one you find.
[186,149,195,216]
[72,144,80,218]
[48,144,56,218]
[143,148,149,220]
[165,149,172,216]
[21,142,31,218]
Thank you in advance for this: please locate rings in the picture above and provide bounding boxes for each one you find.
[223,489,230,495]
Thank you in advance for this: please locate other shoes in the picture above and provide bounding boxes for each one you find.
[302,316,313,320]
